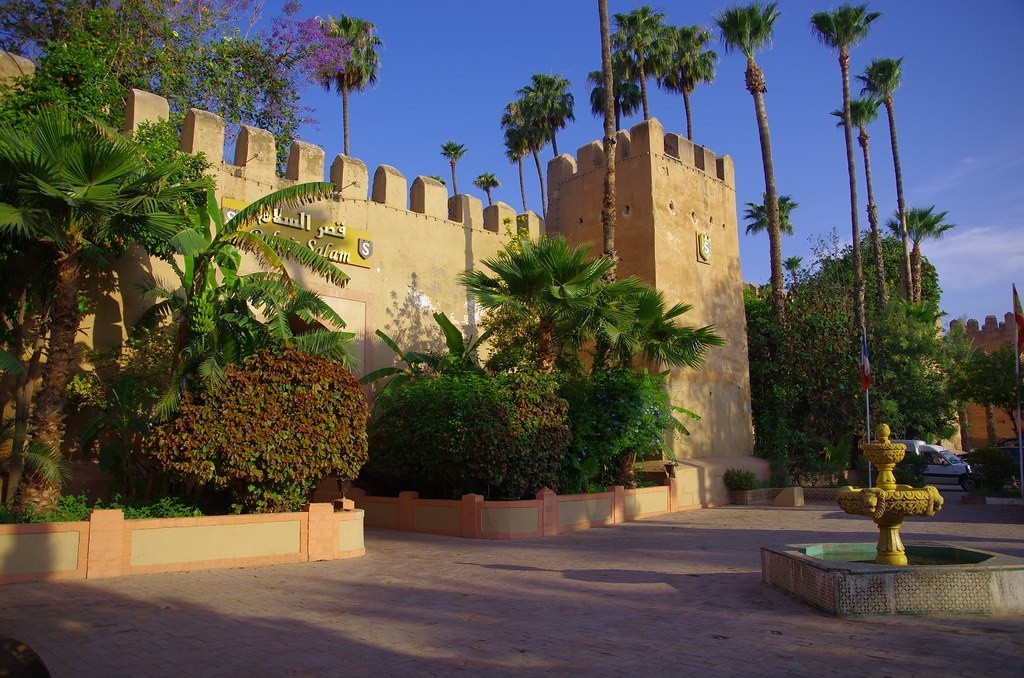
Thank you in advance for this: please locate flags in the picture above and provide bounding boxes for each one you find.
[861,328,871,391]
[1015,287,1024,355]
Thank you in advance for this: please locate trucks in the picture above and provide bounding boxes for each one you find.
[870,440,975,489]
